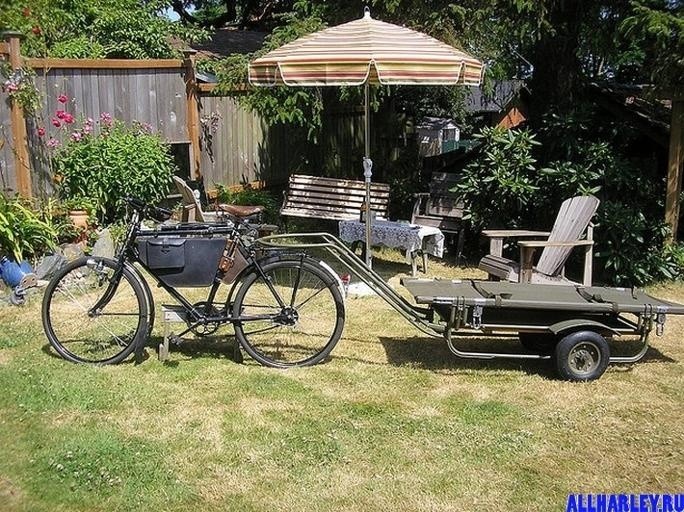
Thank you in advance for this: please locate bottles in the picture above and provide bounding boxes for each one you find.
[359,195,368,223]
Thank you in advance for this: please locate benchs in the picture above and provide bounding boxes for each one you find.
[275,172,390,251]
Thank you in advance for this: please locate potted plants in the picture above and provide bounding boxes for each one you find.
[60,191,101,234]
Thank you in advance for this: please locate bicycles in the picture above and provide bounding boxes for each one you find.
[39,198,351,368]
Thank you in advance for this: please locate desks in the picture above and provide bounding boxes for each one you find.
[337,217,446,280]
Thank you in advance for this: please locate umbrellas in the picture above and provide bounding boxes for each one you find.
[245,6,486,298]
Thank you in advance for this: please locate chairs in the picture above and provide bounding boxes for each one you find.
[410,162,483,269]
[166,170,236,224]
[472,190,597,288]
